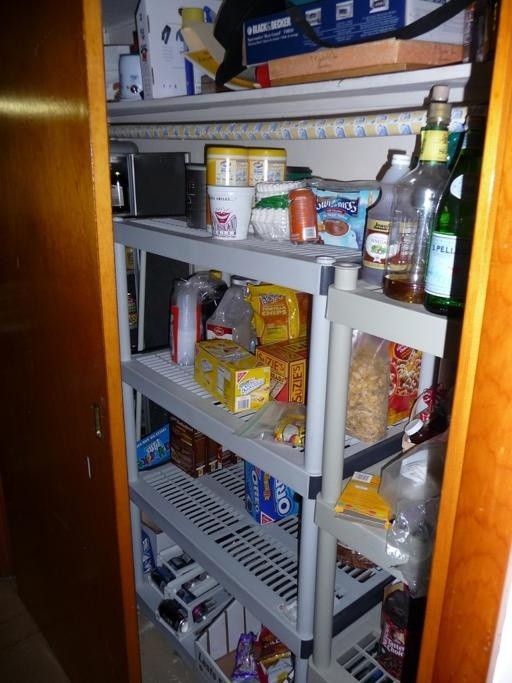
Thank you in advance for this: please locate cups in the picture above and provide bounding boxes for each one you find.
[118,54,145,102]
[206,184,255,241]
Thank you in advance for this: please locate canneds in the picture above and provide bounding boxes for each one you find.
[289,187,318,243]
[151,553,206,633]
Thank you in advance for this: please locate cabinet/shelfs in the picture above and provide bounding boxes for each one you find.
[110,212,448,683]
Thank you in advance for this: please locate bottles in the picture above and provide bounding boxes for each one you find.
[110,161,130,214]
[360,84,478,318]
[184,163,207,229]
[200,269,229,340]
